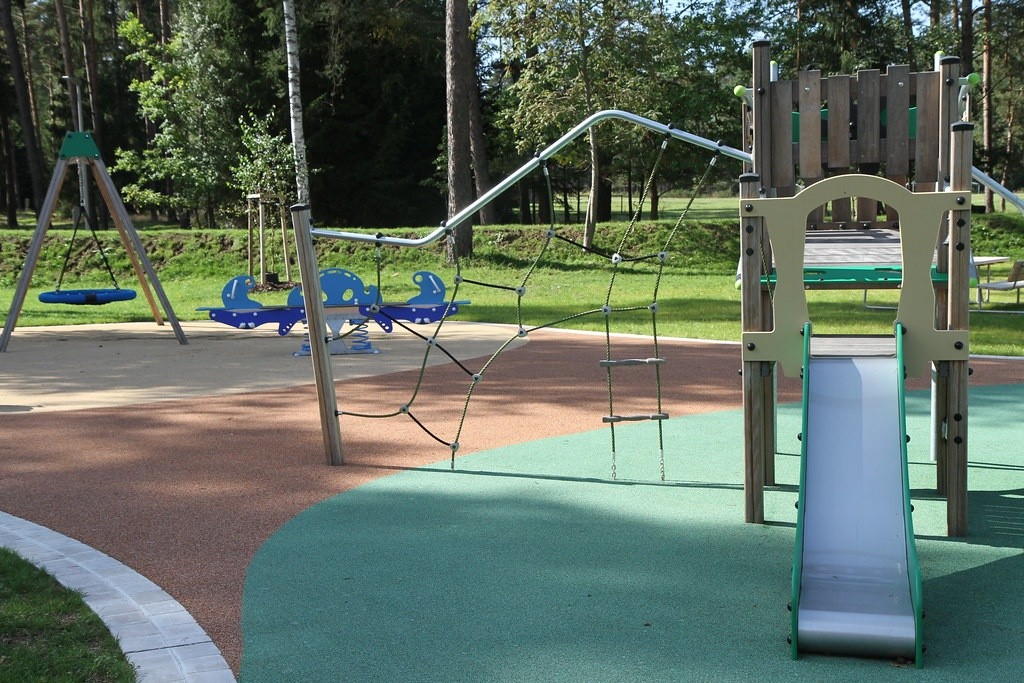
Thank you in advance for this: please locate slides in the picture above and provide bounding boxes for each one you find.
[784,324,924,670]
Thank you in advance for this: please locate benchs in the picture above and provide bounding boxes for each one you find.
[977,261,1024,314]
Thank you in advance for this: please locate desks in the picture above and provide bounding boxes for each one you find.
[967,256,1010,305]
